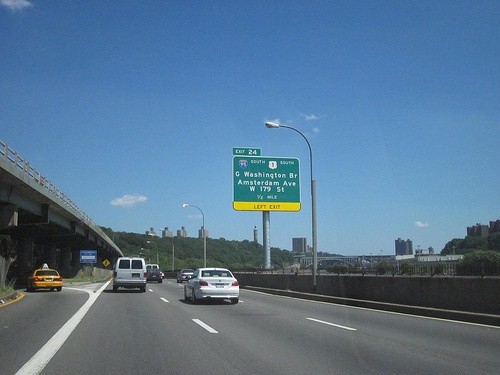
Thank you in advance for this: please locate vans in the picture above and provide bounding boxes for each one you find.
[112,257,147,292]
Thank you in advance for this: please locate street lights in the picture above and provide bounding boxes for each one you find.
[147,240,159,267]
[182,204,206,268]
[159,228,174,272]
[140,248,151,264]
[265,121,318,288]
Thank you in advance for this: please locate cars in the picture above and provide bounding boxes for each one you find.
[26,263,63,292]
[146,263,165,282]
[176,269,195,283]
[184,268,240,304]
